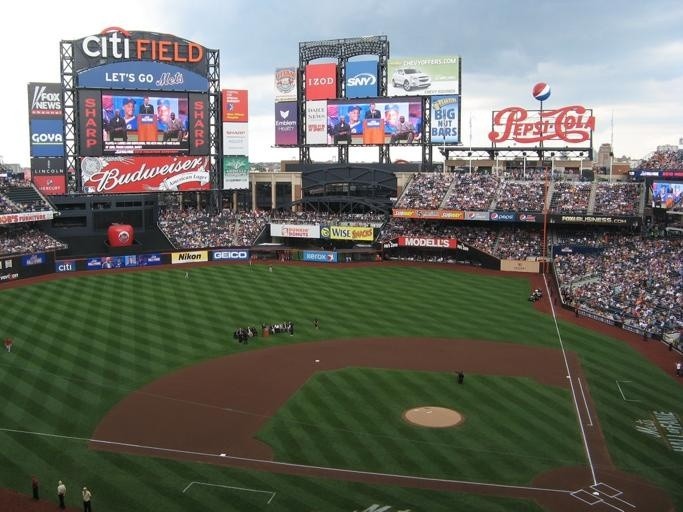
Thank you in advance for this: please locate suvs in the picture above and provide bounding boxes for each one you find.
[391,68,433,91]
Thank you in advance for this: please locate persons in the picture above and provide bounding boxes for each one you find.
[31,475,39,501]
[0,179,63,255]
[81,487,93,512]
[675,360,682,376]
[159,209,390,279]
[4,337,13,352]
[56,480,66,509]
[455,369,464,383]
[103,97,186,141]
[389,167,682,352]
[234,320,295,345]
[312,317,321,330]
[328,103,422,145]
